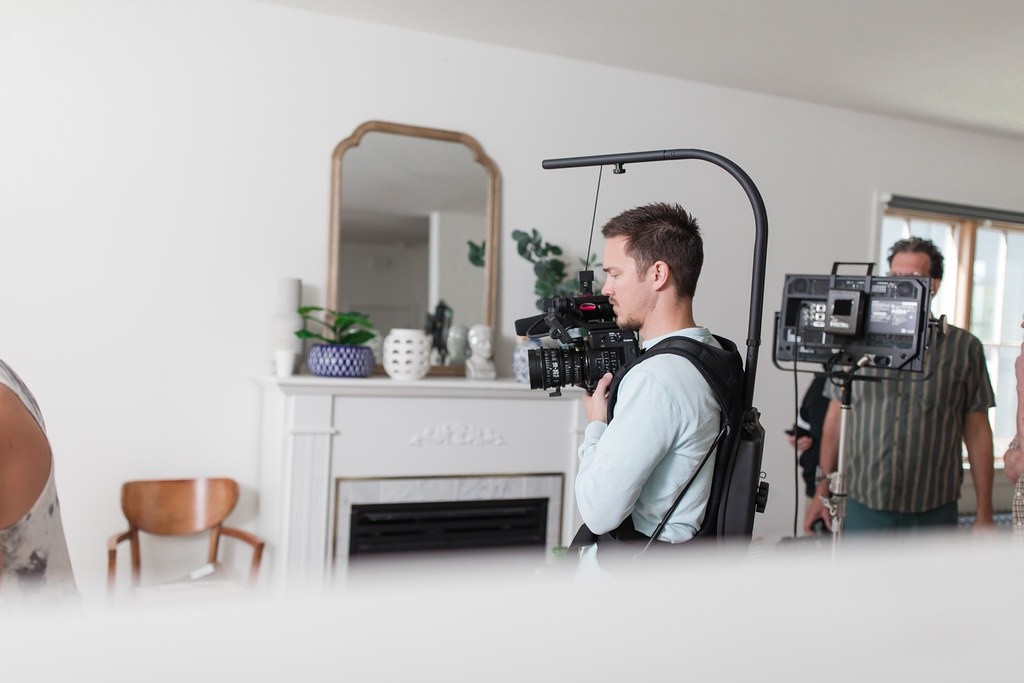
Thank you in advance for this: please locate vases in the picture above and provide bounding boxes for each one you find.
[384,328,431,381]
[274,273,304,375]
[514,336,554,386]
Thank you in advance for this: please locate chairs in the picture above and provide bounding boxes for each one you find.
[107,478,267,592]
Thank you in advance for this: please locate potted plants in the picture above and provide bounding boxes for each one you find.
[507,227,601,351]
[293,305,376,377]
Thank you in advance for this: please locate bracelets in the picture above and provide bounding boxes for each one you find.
[814,466,829,486]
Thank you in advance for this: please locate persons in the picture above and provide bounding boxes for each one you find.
[0,357,78,600]
[445,326,470,367]
[786,370,839,540]
[1003,314,1024,533]
[576,202,723,563]
[805,237,998,534]
[464,325,497,379]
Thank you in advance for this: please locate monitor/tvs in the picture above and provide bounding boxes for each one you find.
[432,301,454,347]
[776,273,933,373]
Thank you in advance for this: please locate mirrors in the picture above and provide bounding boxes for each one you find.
[324,120,504,373]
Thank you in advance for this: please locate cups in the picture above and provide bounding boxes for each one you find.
[383,328,434,381]
